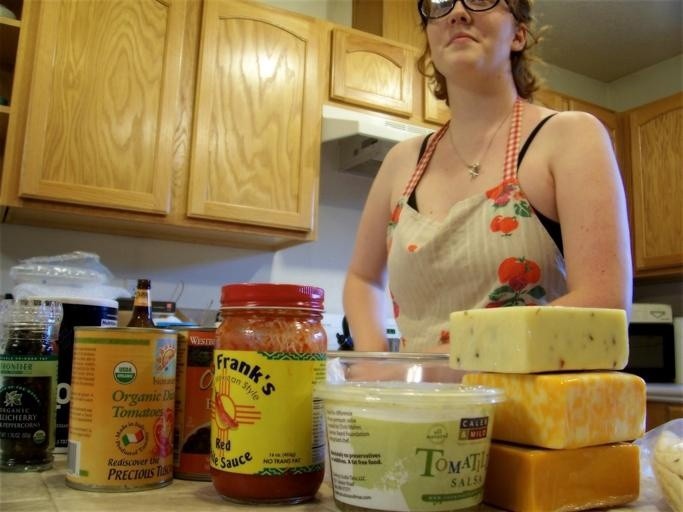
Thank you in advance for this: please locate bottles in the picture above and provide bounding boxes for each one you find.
[126,278,156,328]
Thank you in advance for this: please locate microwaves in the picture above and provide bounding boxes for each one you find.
[615,303,676,384]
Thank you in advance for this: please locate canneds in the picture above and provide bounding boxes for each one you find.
[164,326,216,482]
[64,324,179,493]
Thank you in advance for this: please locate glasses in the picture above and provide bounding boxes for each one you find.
[420,0,518,19]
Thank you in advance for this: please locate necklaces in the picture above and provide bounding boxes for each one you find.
[449,111,513,181]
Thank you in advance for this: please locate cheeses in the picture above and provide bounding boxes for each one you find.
[484,441,640,512]
[461,371,647,449]
[445,303,630,374]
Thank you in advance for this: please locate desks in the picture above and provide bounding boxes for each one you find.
[0,459,681,510]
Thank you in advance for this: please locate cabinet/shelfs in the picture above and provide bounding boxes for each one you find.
[528,84,682,284]
[5,0,326,252]
[326,18,450,173]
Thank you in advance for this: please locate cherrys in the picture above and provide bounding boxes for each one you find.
[486,192,541,307]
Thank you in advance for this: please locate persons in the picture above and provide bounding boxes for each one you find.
[343,0,633,382]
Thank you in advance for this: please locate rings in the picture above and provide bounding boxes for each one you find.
[345,365,351,379]
